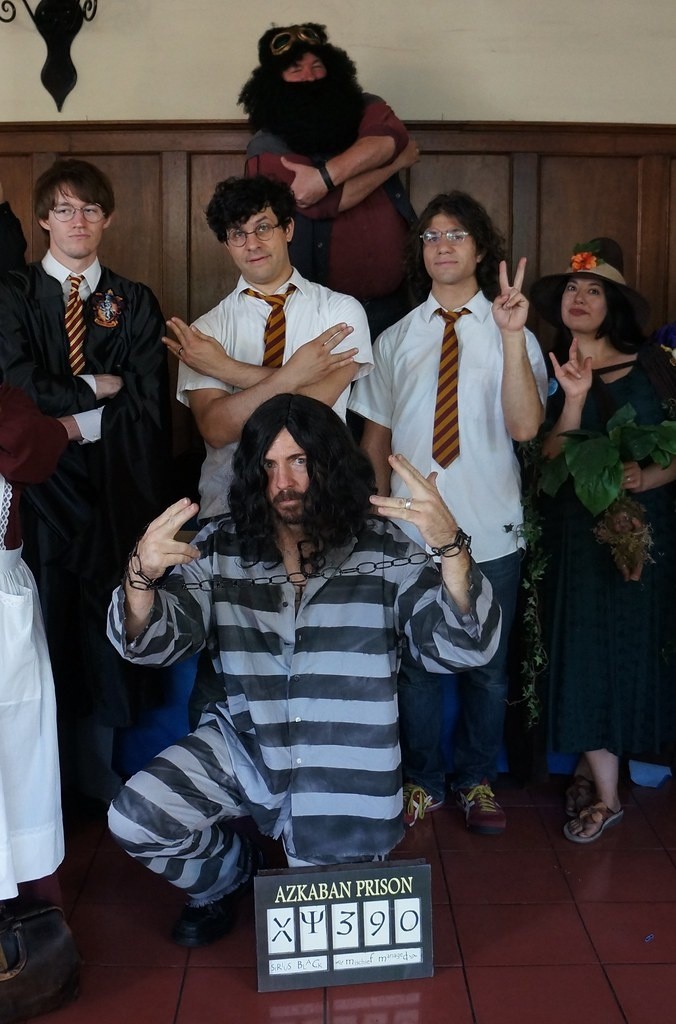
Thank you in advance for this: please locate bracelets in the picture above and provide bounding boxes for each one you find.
[318,163,336,191]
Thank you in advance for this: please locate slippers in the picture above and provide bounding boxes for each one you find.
[563,799,624,844]
[562,775,596,819]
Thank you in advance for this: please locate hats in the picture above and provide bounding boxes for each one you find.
[530,238,652,335]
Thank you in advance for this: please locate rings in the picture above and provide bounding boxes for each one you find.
[179,347,184,355]
[406,497,414,509]
[626,476,631,481]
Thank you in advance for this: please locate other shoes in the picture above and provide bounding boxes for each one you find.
[170,836,257,947]
[60,787,106,833]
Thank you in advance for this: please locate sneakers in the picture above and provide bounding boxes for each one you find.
[400,782,443,827]
[451,777,506,836]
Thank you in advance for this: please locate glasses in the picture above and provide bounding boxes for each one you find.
[419,226,471,246]
[224,221,280,248]
[49,202,107,224]
[269,25,320,57]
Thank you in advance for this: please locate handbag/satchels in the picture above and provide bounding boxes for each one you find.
[0,898,82,1024]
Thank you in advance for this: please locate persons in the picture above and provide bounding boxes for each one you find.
[0,178,33,382]
[100,393,504,951]
[508,237,676,841]
[21,160,179,815]
[156,173,375,531]
[589,495,649,583]
[344,189,551,843]
[237,21,434,342]
[0,377,82,1023]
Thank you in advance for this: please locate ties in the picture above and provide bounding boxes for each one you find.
[243,282,298,369]
[63,275,86,377]
[432,308,472,469]
